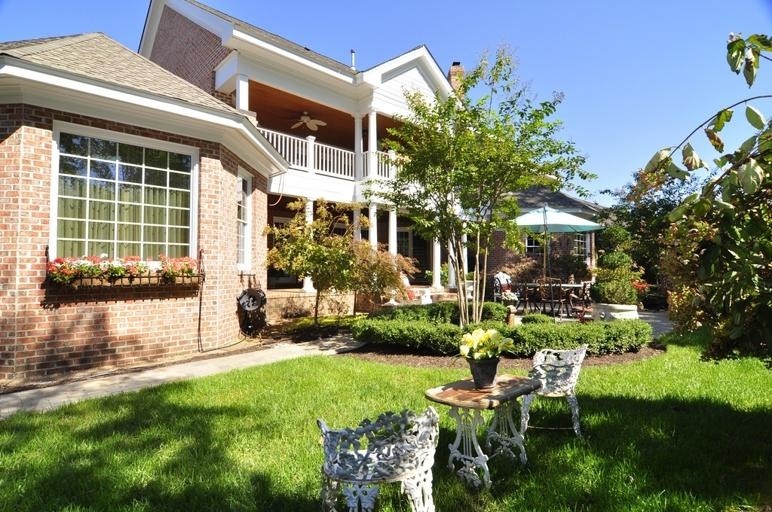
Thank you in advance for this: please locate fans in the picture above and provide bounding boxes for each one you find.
[289,111,328,132]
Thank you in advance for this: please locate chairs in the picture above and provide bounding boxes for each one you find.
[518,343,589,441]
[317,405,440,512]
[493,271,595,324]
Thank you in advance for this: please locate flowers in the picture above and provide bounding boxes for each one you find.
[44,254,199,287]
[456,327,518,360]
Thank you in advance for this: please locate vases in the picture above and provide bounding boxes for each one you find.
[466,357,502,390]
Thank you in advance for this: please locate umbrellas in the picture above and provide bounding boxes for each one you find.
[495,204,604,283]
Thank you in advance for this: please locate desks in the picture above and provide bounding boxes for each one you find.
[423,373,543,492]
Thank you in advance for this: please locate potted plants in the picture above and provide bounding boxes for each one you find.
[594,280,641,323]
